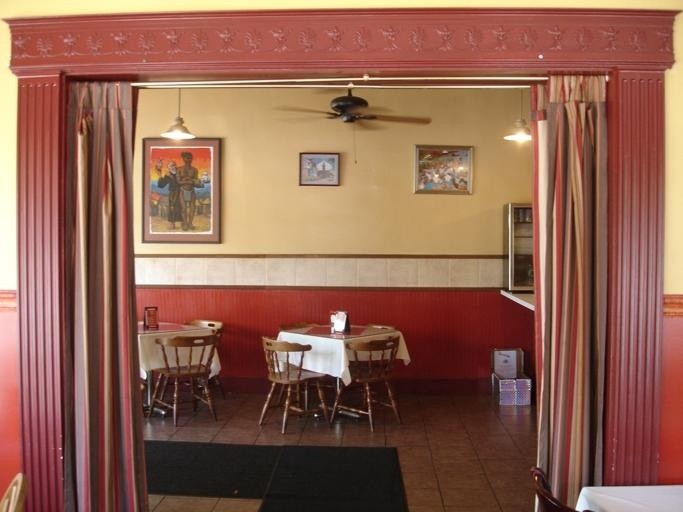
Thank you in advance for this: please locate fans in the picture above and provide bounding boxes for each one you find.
[273,87,432,124]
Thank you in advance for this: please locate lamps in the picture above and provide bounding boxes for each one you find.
[503,89,532,142]
[160,88,196,140]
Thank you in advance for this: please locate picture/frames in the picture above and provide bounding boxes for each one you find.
[413,142,474,195]
[298,152,340,186]
[141,137,222,244]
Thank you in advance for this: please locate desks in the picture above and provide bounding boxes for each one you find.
[499,288,536,313]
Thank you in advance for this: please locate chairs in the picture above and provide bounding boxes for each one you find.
[281,323,336,392]
[258,336,333,434]
[491,347,532,406]
[0,472,27,512]
[329,335,403,425]
[528,464,579,512]
[146,335,219,427]
[181,319,226,401]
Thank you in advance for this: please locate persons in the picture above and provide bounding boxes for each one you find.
[158,160,182,228]
[175,152,200,230]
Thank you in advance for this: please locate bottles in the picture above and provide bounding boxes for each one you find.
[513,208,531,222]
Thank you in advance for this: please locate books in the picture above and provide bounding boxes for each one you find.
[492,348,524,379]
